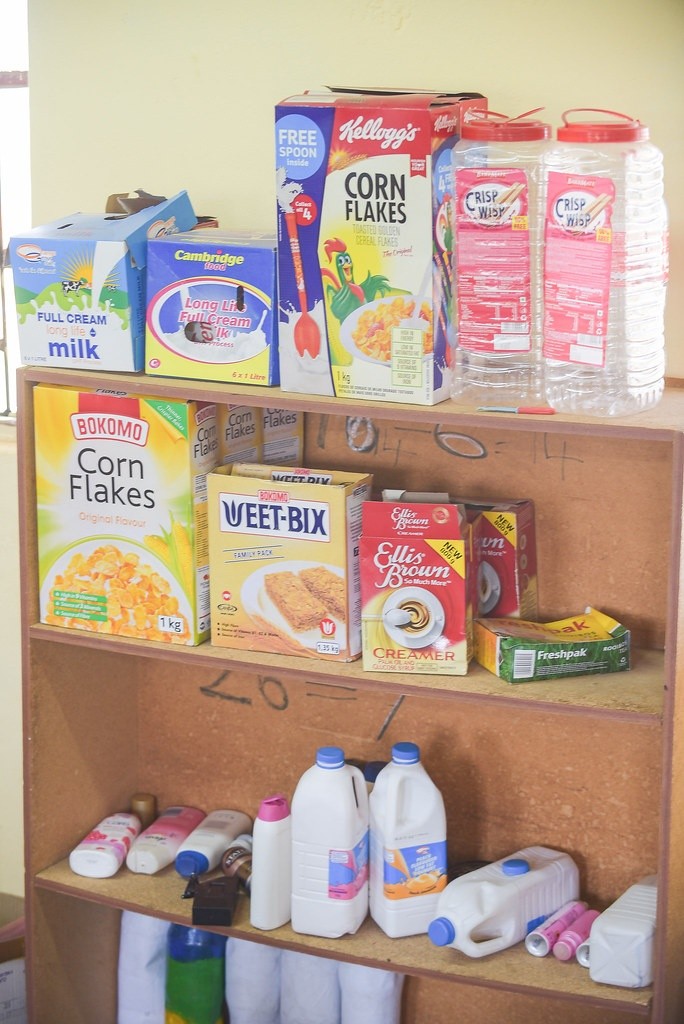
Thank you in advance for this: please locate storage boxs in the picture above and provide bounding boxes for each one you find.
[275,94,461,406]
[145,230,281,388]
[216,403,262,466]
[4,191,198,372]
[263,407,304,470]
[473,606,630,685]
[33,383,217,647]
[450,496,538,621]
[105,188,219,231]
[325,82,487,167]
[358,498,473,674]
[382,489,484,619]
[206,462,374,663]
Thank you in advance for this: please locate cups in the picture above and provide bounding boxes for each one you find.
[482,573,491,603]
[394,597,434,637]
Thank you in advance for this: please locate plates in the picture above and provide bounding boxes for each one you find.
[338,296,434,366]
[40,534,192,644]
[381,587,445,649]
[481,560,500,616]
[240,561,347,650]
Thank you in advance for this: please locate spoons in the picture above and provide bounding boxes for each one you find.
[362,610,409,624]
[285,214,320,358]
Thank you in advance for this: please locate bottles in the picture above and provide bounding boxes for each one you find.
[363,761,391,795]
[590,873,660,988]
[176,809,252,877]
[367,742,447,937]
[575,937,590,968]
[544,109,671,417]
[127,806,203,873]
[449,107,550,405]
[69,811,141,877]
[251,796,292,931]
[429,846,581,958]
[291,746,370,939]
[553,910,600,960]
[525,901,588,957]
[221,847,252,891]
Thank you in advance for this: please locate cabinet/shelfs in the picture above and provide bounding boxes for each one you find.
[15,366,684,1024]
